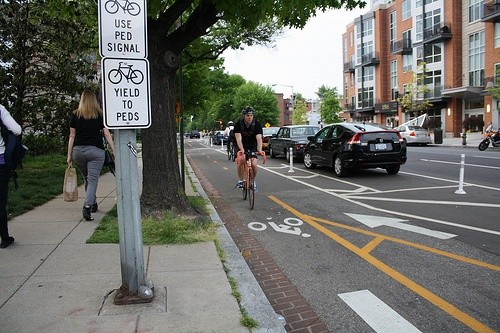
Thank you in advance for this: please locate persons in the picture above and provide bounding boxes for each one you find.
[0,104,22,249]
[223,120,237,158]
[66,90,115,221]
[233,105,265,193]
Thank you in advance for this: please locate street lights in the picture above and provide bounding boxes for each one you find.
[271,83,296,123]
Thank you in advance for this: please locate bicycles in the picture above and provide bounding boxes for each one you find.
[227,143,237,161]
[241,151,267,209]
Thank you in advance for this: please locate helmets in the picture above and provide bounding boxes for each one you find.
[228,121,233,125]
[242,106,255,116]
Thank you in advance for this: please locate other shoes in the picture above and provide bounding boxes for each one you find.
[0,236,14,248]
[250,183,257,191]
[83,205,94,221]
[237,181,245,188]
[91,203,97,213]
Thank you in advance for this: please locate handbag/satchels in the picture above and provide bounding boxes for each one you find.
[104,149,115,170]
[3,134,29,173]
[63,160,78,201]
[230,131,235,137]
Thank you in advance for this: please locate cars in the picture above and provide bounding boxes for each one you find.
[209,131,230,143]
[303,122,409,177]
[393,125,432,145]
[262,127,280,154]
[267,124,323,163]
[190,130,200,139]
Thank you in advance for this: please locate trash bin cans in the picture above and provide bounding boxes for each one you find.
[433,129,443,144]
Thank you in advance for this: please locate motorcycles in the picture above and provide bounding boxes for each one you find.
[478,123,500,151]
